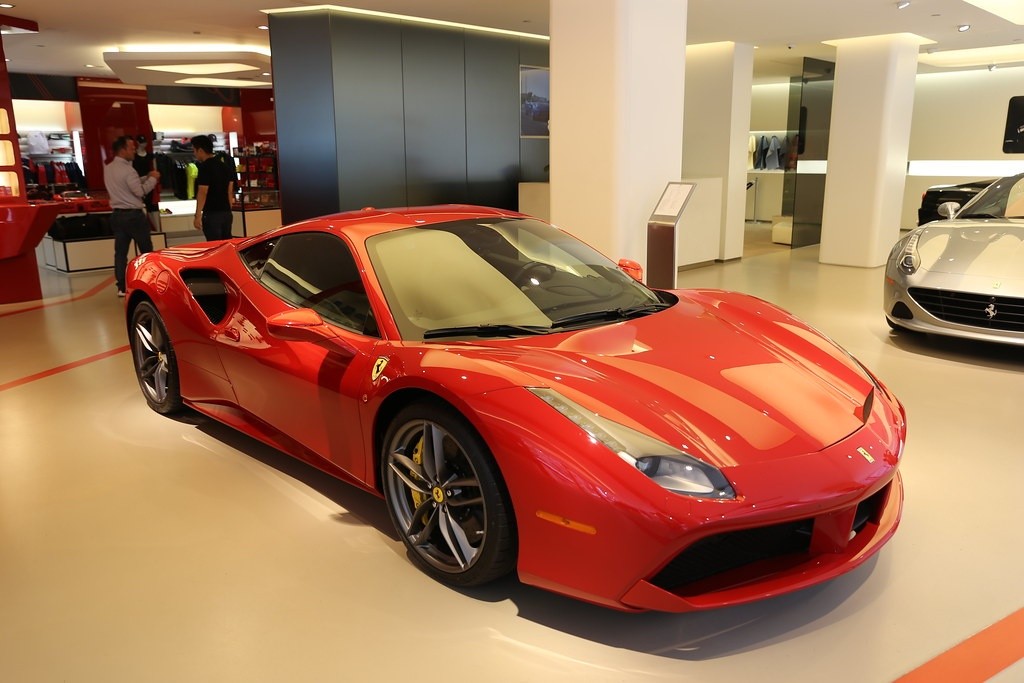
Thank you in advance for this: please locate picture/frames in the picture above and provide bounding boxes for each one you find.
[519,64,549,138]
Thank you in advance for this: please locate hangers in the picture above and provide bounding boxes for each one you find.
[159,150,190,169]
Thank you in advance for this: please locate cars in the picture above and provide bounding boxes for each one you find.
[524,96,549,120]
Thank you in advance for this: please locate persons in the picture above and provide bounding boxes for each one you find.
[190,135,234,241]
[129,135,162,234]
[102,135,161,297]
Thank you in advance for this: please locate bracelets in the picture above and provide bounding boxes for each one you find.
[195,214,201,218]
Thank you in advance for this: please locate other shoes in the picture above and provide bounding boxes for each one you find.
[117,287,126,296]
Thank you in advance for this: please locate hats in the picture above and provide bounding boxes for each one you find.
[137,135,147,143]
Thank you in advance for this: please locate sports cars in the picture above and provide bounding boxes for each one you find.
[122,204,909,613]
[882,172,1024,347]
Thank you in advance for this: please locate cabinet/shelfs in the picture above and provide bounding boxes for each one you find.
[233,153,278,203]
